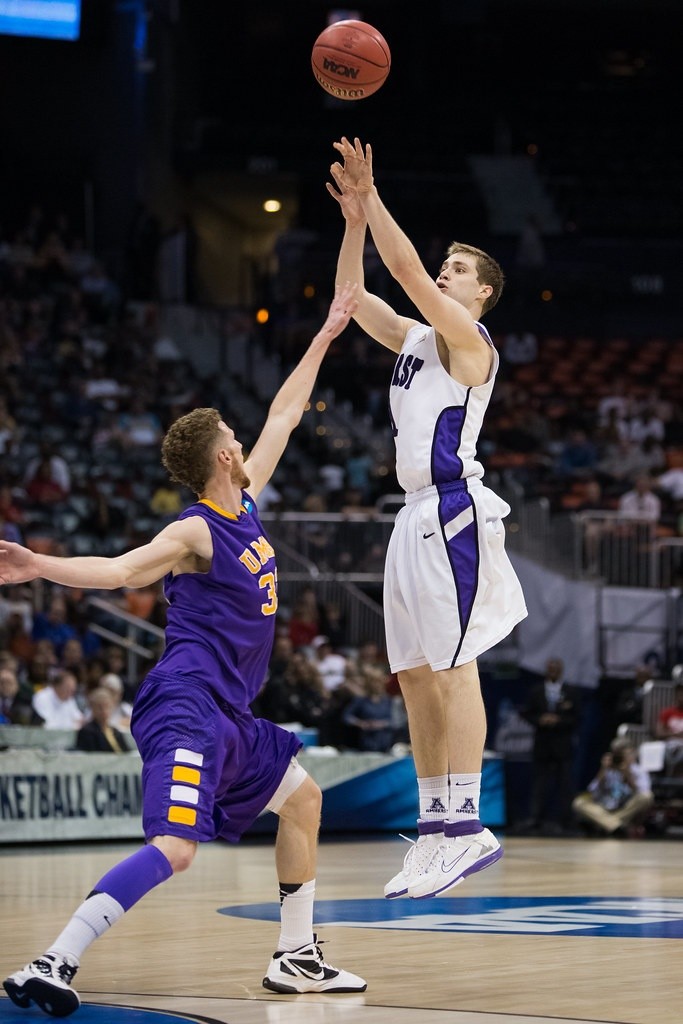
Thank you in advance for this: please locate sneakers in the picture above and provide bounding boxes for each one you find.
[2,948,82,1017]
[262,932,367,994]
[384,818,450,898]
[408,820,504,900]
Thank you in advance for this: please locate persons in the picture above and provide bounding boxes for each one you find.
[0,114,683,832]
[325,137,529,901]
[0,280,367,1018]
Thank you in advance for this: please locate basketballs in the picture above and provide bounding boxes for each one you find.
[311,19,392,100]
